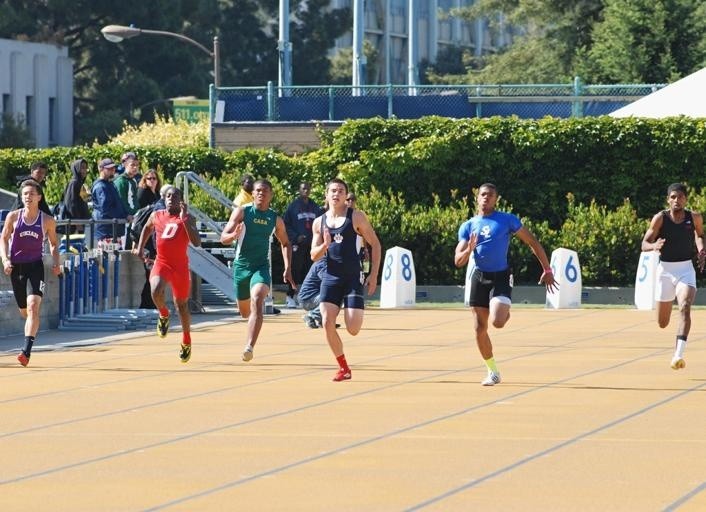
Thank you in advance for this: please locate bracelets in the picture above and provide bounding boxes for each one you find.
[543,269,552,273]
[180,216,189,223]
[1,258,9,263]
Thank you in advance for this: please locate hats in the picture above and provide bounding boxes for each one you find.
[99,158,117,169]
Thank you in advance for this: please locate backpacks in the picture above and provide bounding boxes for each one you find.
[130,203,164,251]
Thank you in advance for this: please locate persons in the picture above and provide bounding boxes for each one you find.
[18,153,175,308]
[136,188,200,362]
[221,175,381,383]
[0,178,62,367]
[641,183,706,369]
[454,182,559,385]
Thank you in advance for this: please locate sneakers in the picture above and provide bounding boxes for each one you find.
[482,370,500,386]
[332,368,351,381]
[303,316,319,328]
[178,343,191,363]
[285,295,295,308]
[670,357,685,370]
[242,349,253,362]
[17,353,29,367]
[157,314,170,339]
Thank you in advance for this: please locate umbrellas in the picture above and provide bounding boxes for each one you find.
[608,65,706,118]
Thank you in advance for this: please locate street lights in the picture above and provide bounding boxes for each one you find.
[100,20,220,87]
[132,92,197,111]
